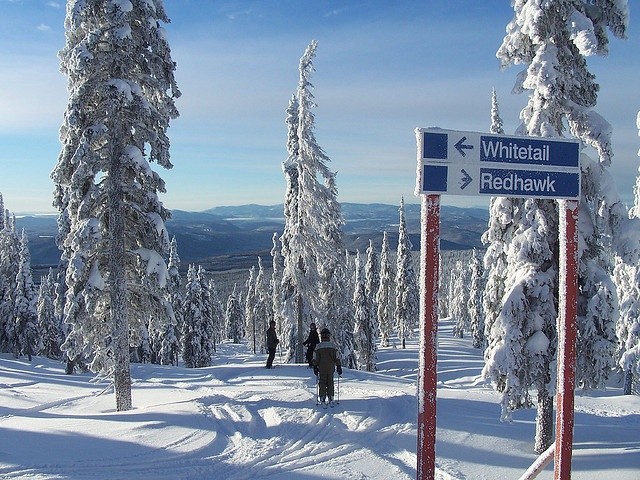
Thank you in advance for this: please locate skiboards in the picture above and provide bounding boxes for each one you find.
[321,401,334,409]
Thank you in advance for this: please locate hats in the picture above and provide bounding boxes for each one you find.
[320,328,331,334]
[310,323,316,329]
[270,320,276,326]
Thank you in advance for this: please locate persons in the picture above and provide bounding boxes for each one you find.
[265,321,279,369]
[313,328,342,404]
[303,323,320,370]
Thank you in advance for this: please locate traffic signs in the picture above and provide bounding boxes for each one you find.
[422,128,581,168]
[421,162,581,200]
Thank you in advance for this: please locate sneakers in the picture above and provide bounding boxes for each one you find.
[328,395,333,402]
[320,397,326,402]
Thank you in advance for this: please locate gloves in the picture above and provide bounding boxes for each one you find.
[314,365,319,376]
[337,365,342,375]
